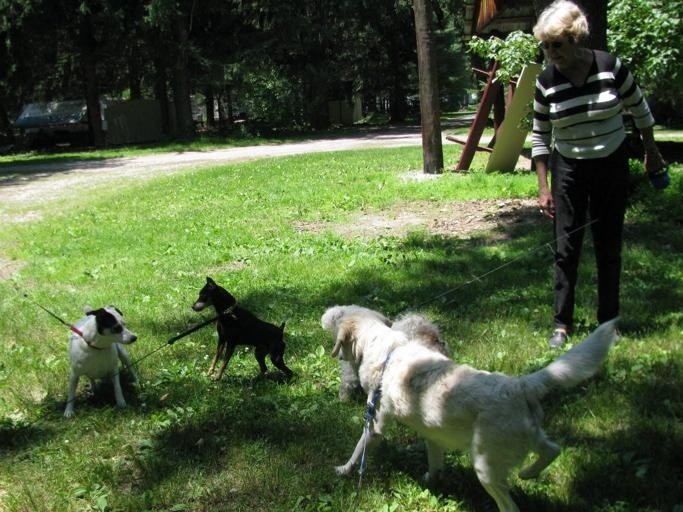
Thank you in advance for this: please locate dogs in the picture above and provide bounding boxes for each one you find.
[191,277,293,384]
[320,306,448,403]
[331,306,621,512]
[64,304,139,417]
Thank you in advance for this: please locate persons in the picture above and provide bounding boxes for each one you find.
[529,1,670,351]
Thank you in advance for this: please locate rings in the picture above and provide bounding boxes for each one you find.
[539,208,544,213]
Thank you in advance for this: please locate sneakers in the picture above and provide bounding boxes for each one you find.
[549,327,567,348]
